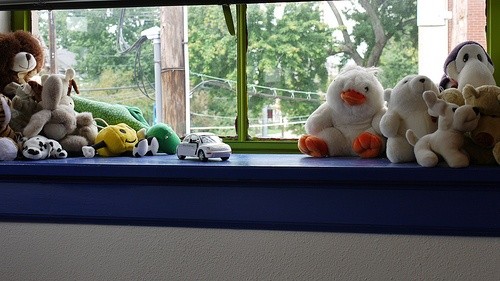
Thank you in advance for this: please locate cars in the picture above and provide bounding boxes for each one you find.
[177,133,232,162]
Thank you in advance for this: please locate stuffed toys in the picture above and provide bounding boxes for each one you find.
[0,30,46,161]
[443,41,496,91]
[406,90,480,167]
[438,85,500,165]
[298,64,388,157]
[70,94,180,154]
[380,74,439,163]
[91,118,159,157]
[0,68,97,159]
